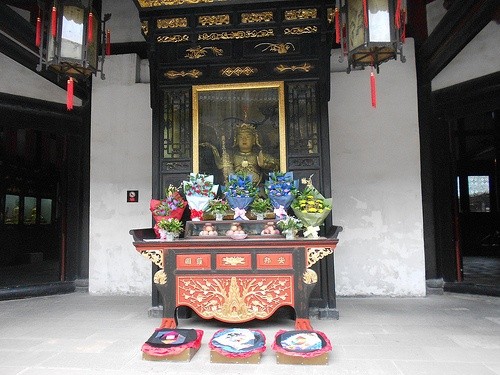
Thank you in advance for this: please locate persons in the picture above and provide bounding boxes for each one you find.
[5,199,47,225]
[198,123,280,199]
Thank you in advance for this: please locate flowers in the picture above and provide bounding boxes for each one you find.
[276,214,302,231]
[153,187,184,216]
[184,173,217,196]
[265,172,299,196]
[159,218,184,234]
[224,175,256,196]
[294,187,331,213]
[210,198,229,213]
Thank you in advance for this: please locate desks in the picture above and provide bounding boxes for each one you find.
[129,225,344,331]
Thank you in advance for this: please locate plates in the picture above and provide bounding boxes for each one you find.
[261,234,281,238]
[199,235,217,239]
[226,234,247,239]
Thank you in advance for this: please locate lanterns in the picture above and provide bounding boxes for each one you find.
[333,0,407,107]
[36,0,113,109]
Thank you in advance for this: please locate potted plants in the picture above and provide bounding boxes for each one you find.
[251,198,271,221]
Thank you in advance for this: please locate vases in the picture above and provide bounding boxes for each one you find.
[216,213,223,222]
[166,232,175,241]
[285,229,294,238]
